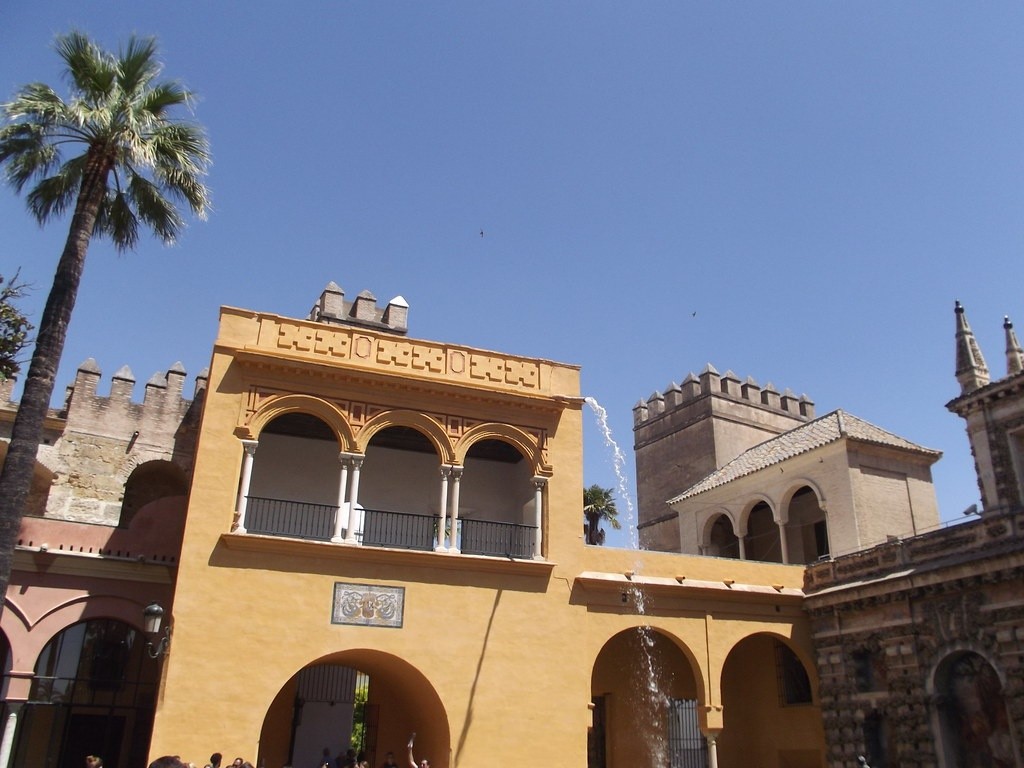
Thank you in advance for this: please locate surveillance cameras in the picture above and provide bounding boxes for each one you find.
[962,504,976,516]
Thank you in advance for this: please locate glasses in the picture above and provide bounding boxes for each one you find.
[420,762,425,766]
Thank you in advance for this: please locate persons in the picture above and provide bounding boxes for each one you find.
[408,741,430,768]
[203,753,255,768]
[383,752,398,768]
[86,756,103,768]
[148,755,189,768]
[319,748,370,768]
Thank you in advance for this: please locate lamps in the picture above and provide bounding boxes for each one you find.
[144,601,173,660]
